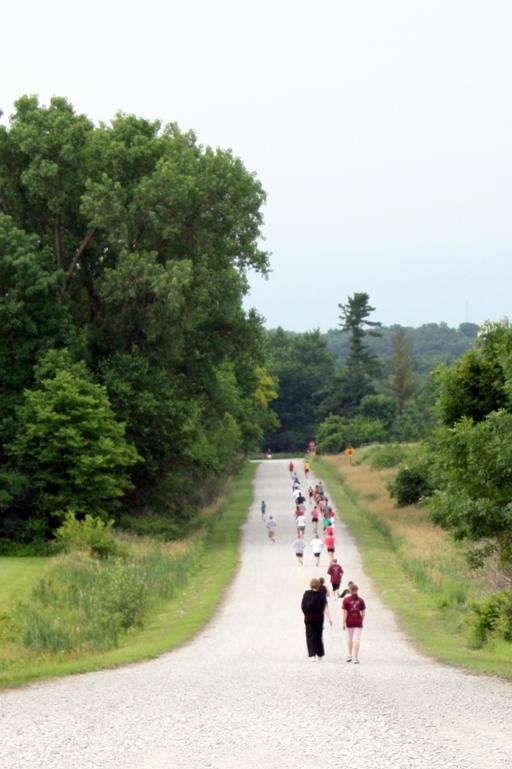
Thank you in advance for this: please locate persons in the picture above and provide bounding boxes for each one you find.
[260,460,366,664]
